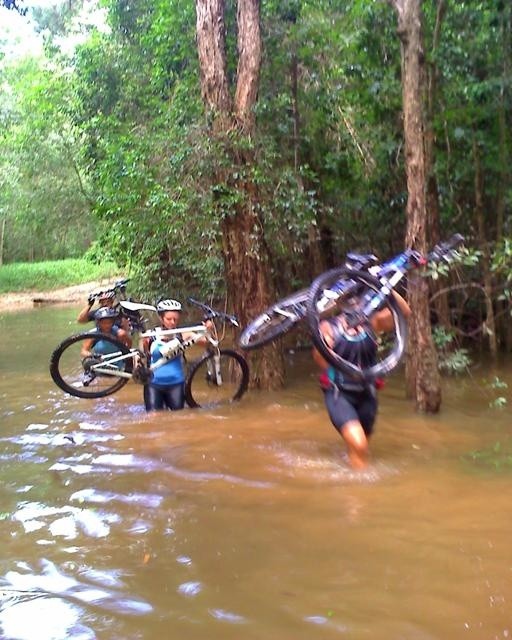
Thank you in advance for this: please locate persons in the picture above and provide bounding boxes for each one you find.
[133,298,215,411]
[78,289,132,344]
[310,286,412,474]
[79,307,133,379]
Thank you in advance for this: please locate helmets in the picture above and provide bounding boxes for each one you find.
[157,299,182,312]
[94,307,116,321]
[98,290,115,303]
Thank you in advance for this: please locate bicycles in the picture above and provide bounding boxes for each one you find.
[237,232,464,379]
[50,299,249,408]
[88,278,156,335]
